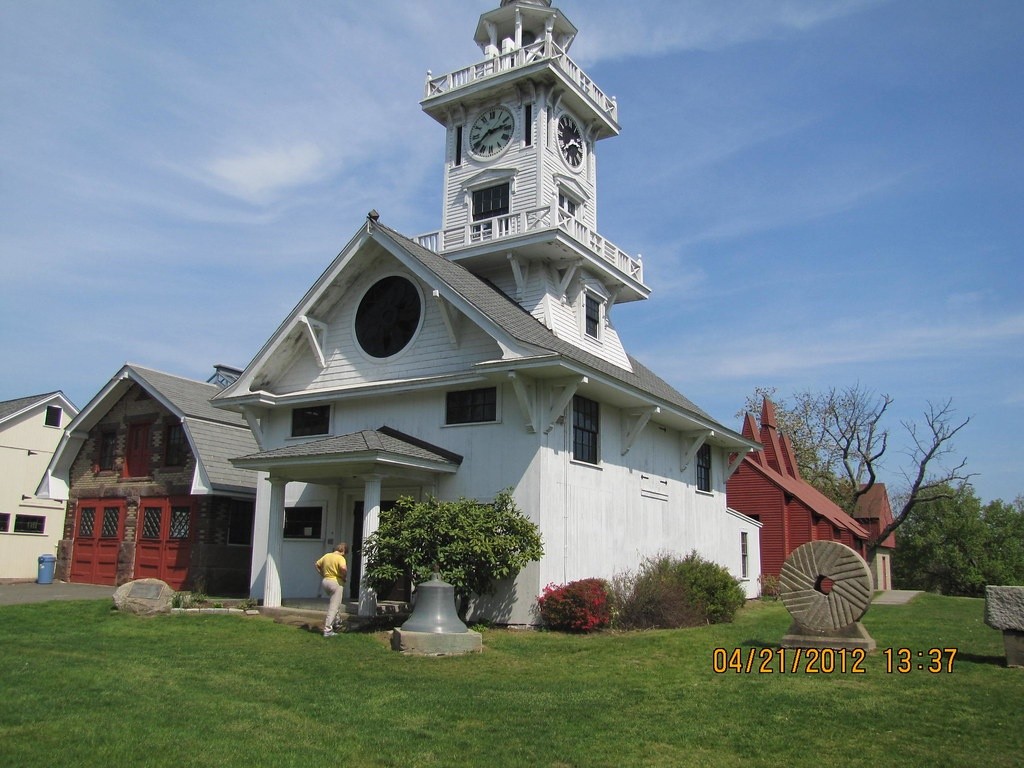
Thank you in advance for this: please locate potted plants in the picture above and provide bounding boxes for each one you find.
[361,489,546,616]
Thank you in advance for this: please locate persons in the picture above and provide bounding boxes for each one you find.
[315,543,349,636]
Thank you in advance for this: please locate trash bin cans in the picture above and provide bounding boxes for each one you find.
[36,554,56,584]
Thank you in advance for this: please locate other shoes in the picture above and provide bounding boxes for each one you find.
[334,619,343,628]
[324,630,339,637]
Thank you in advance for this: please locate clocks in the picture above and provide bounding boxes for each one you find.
[555,111,586,175]
[466,104,518,163]
